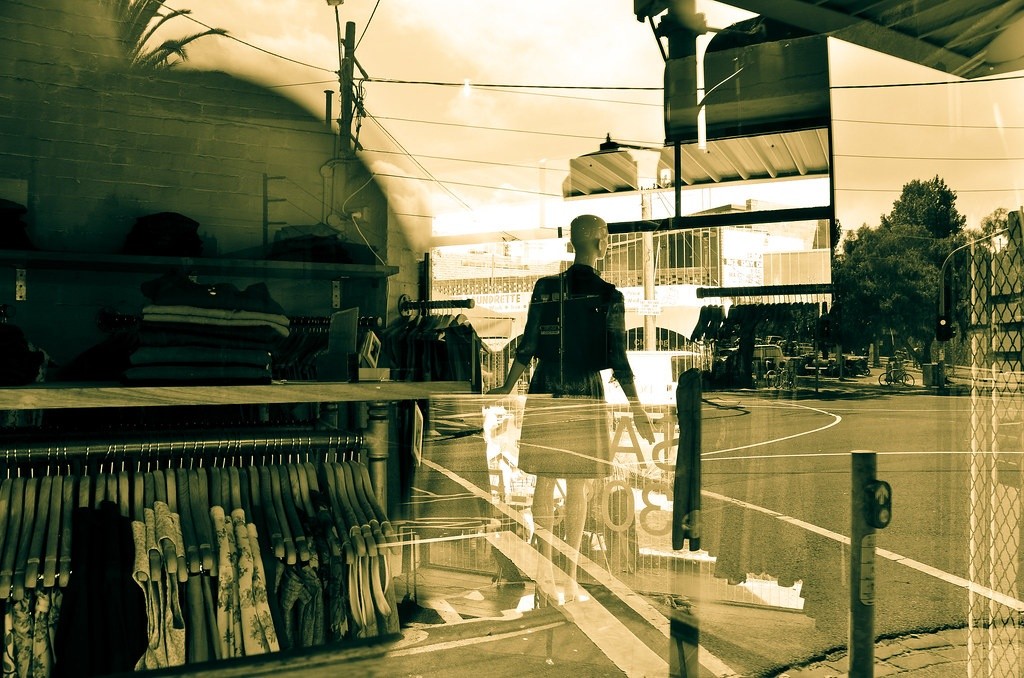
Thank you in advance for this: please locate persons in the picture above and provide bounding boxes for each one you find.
[481,215,655,605]
[885,361,892,385]
[893,357,901,384]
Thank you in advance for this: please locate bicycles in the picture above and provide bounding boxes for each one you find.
[880,348,914,389]
[766,358,798,391]
[835,356,870,379]
